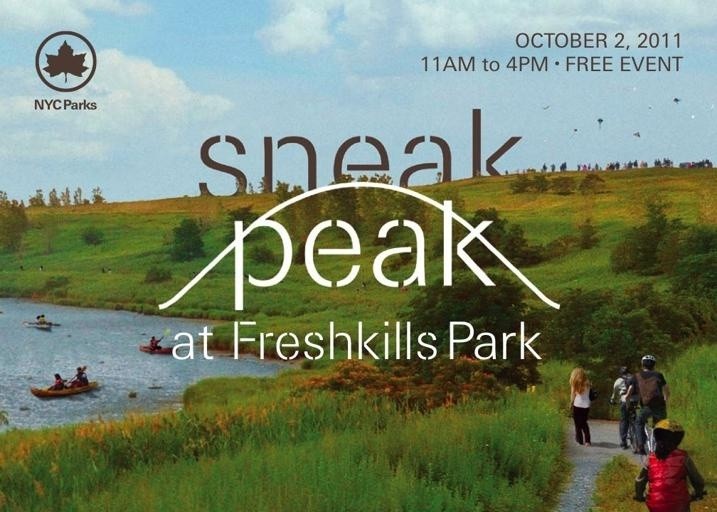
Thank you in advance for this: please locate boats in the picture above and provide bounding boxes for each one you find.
[30,380,98,398]
[139,345,178,354]
[23,321,52,330]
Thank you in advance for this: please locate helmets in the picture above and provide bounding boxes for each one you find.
[654,419,685,440]
[642,355,656,368]
[619,366,628,374]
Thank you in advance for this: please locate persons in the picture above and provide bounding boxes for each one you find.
[148,336,162,350]
[610,366,642,449]
[623,354,671,455]
[68,367,88,388]
[568,367,594,448]
[35,316,40,323]
[49,374,64,390]
[634,419,704,512]
[39,314,46,324]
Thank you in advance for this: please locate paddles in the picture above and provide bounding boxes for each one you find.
[160,329,171,340]
[65,366,86,384]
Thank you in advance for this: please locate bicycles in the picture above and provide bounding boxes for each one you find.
[626,404,657,455]
[633,490,707,503]
[608,399,640,455]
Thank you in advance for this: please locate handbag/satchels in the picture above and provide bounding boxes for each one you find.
[589,388,599,401]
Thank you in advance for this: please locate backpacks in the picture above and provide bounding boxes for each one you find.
[624,377,635,393]
[639,377,658,405]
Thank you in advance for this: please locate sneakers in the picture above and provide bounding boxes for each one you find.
[584,442,591,448]
[634,445,645,455]
[620,441,627,449]
